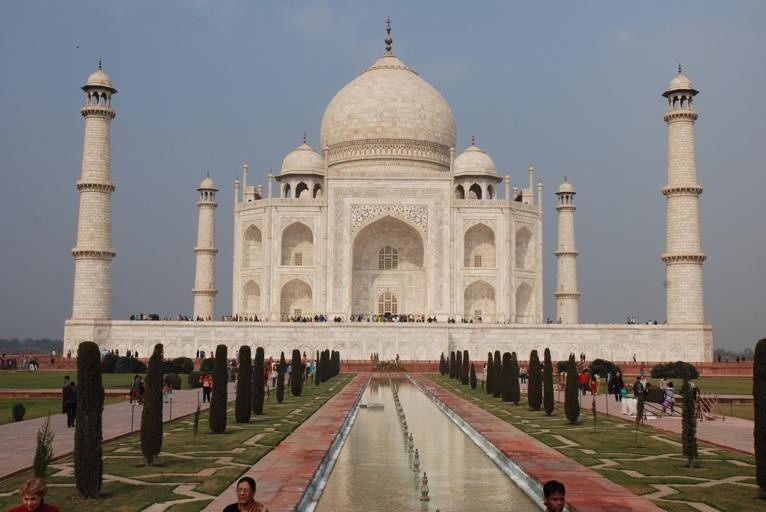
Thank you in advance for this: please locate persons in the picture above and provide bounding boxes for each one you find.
[520,365,527,384]
[130,375,145,405]
[608,371,623,402]
[264,360,316,385]
[130,313,483,324]
[162,381,169,403]
[196,350,213,358]
[621,375,674,415]
[541,479,566,512]
[569,352,585,361]
[633,353,637,363]
[222,477,268,512]
[627,316,657,325]
[62,376,77,426]
[559,367,597,395]
[199,373,212,403]
[718,352,745,362]
[2,348,71,376]
[102,349,131,359]
[4,477,60,511]
[547,317,562,323]
